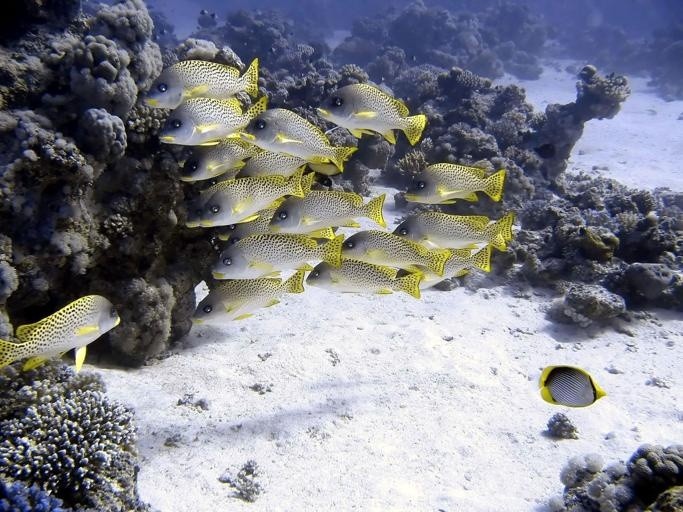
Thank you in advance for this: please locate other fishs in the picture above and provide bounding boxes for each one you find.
[143,57,516,326]
